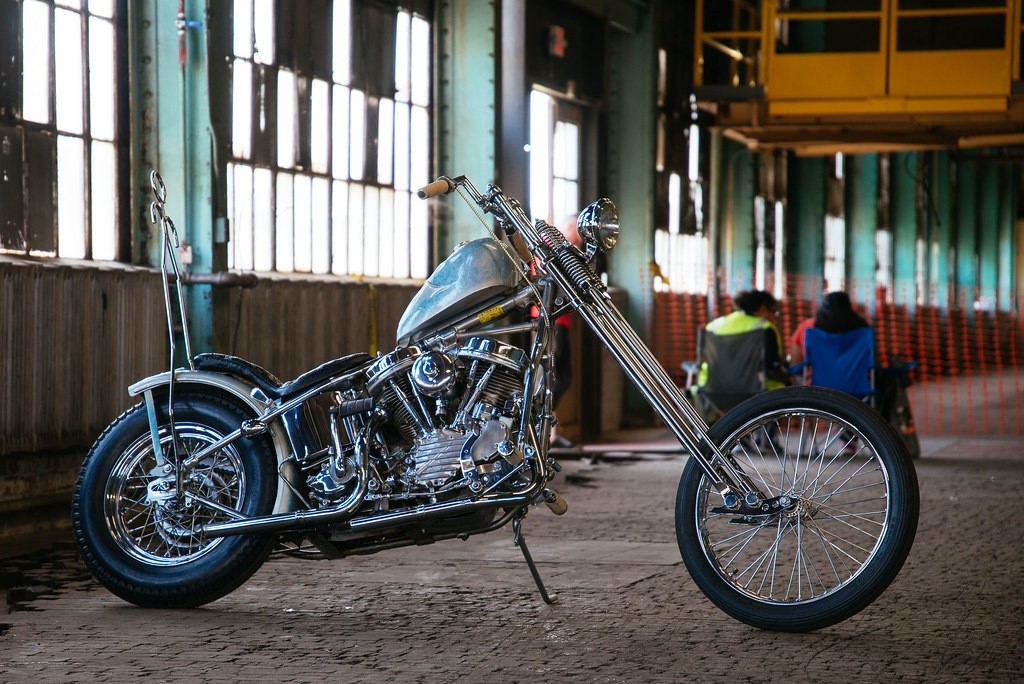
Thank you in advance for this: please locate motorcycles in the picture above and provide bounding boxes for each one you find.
[69,176,924,633]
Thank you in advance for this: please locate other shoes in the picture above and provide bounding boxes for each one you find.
[840,440,857,456]
[757,440,782,455]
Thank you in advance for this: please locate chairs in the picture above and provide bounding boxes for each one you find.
[799,327,915,457]
[681,324,793,457]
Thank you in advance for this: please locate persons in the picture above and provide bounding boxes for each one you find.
[530,221,588,448]
[696,289,882,457]
